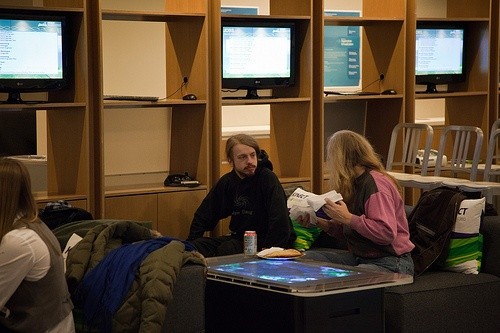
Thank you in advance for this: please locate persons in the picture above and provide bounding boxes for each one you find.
[294,127,415,278]
[0,156,75,333]
[182,131,298,260]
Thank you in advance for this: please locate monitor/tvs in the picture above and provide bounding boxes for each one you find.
[416,24,469,95]
[0,12,65,103]
[222,21,297,98]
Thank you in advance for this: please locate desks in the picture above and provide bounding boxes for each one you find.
[206,255,413,297]
[388,170,500,203]
[454,163,500,175]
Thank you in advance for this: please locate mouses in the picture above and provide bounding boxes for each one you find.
[183,94,197,100]
[381,88,397,95]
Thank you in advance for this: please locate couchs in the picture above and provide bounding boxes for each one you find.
[51,206,500,333]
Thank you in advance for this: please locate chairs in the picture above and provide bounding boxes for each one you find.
[385,123,434,175]
[435,125,484,182]
[482,129,500,205]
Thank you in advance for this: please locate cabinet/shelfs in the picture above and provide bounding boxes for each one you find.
[0,0,500,241]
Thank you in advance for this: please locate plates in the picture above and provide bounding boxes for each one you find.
[258,248,304,260]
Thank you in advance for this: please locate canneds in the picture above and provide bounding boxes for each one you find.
[244,231,257,256]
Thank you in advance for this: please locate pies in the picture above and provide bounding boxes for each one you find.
[263,249,303,258]
